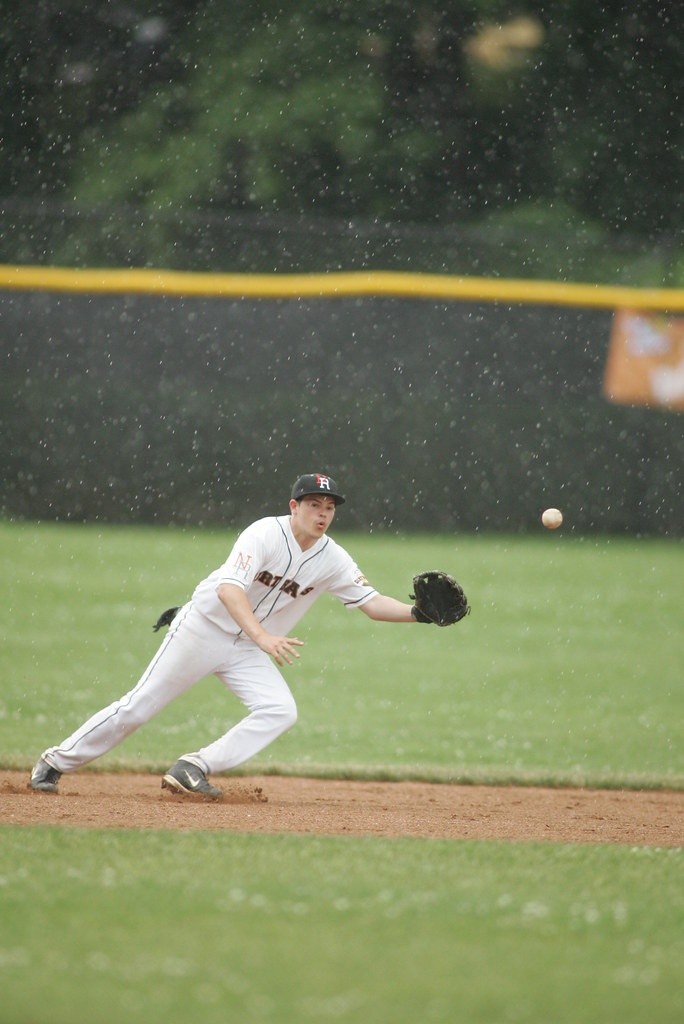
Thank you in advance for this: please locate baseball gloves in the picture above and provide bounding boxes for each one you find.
[410,571,467,628]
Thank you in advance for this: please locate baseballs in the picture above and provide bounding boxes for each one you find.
[542,507,563,530]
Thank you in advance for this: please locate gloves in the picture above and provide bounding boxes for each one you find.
[153,607,180,633]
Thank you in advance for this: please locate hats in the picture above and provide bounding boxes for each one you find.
[291,473,345,505]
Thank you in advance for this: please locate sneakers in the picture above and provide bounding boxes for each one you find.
[160,760,223,799]
[30,757,63,794]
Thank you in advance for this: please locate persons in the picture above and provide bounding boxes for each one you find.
[31,474,472,798]
